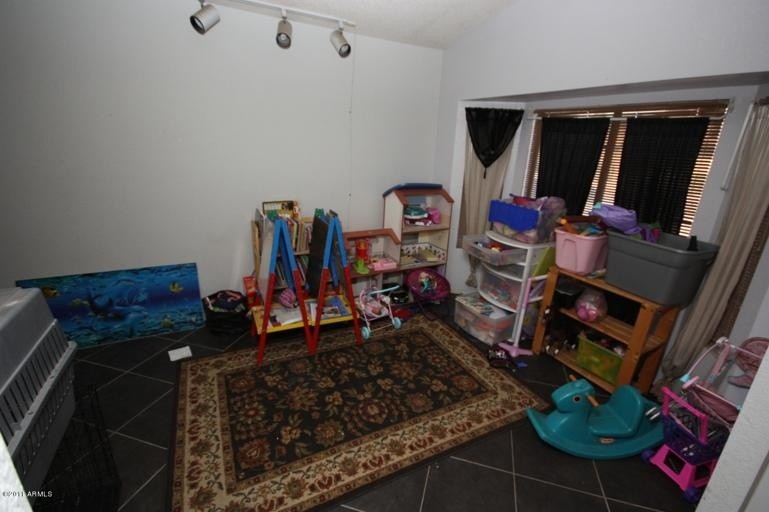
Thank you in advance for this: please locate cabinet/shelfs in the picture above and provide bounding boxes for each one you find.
[454,231,722,401]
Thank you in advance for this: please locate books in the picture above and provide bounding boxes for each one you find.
[254,212,332,286]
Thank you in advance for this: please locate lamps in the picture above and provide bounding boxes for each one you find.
[190,0,355,59]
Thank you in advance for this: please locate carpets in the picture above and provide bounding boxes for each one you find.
[163,311,552,512]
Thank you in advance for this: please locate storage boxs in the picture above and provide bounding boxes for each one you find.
[490,196,721,308]
[0,288,78,495]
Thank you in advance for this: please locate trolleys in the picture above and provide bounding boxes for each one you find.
[354,281,405,338]
